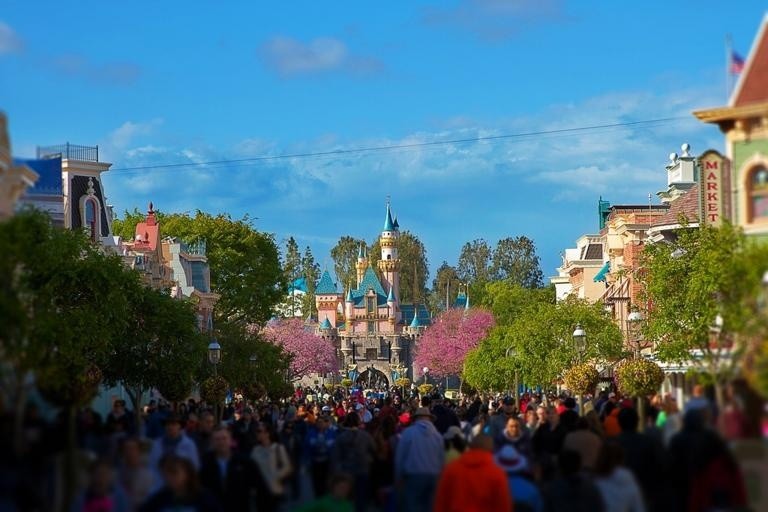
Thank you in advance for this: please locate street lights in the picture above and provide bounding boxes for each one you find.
[343,354,429,411]
[572,319,588,417]
[626,305,649,437]
[506,345,523,415]
[249,354,259,384]
[207,335,221,430]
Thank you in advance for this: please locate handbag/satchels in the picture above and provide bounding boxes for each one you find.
[275,443,296,487]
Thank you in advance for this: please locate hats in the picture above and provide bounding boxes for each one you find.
[503,398,514,405]
[373,408,380,412]
[354,404,363,410]
[379,413,396,434]
[321,406,329,412]
[410,406,437,422]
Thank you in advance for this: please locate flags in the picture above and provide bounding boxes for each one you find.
[730,47,746,73]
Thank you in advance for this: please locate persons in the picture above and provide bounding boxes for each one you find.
[0,382,750,512]
[366,364,377,388]
